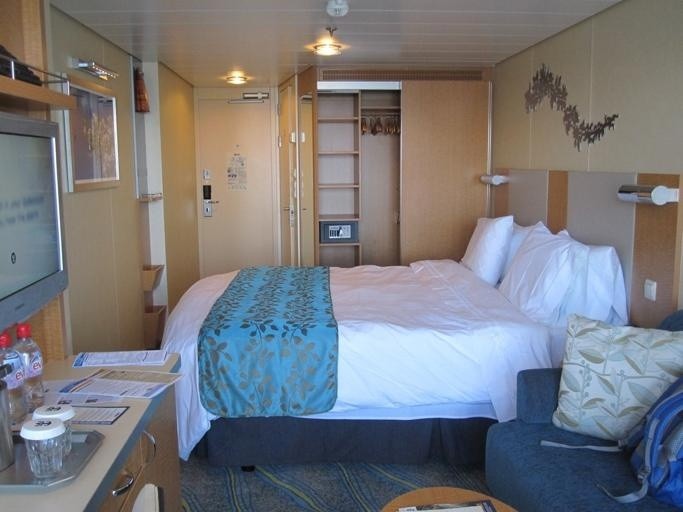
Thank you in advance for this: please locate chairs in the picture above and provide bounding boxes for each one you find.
[489,309,683,510]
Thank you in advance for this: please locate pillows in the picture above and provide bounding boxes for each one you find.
[461,211,619,335]
[551,312,683,440]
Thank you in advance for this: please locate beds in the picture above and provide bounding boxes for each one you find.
[170,166,683,465]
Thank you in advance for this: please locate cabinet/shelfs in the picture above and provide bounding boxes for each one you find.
[298,80,494,266]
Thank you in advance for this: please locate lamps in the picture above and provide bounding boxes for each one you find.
[328,1,349,20]
[479,174,507,186]
[617,184,678,209]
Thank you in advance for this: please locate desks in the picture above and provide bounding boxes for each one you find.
[1,350,183,512]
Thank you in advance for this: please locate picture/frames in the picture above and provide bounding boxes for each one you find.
[61,72,123,194]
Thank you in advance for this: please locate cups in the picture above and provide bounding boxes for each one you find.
[21,419,66,479]
[31,404,76,454]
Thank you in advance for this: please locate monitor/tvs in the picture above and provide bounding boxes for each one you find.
[0,111,69,334]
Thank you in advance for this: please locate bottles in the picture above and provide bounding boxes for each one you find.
[0,331,29,426]
[14,322,45,413]
